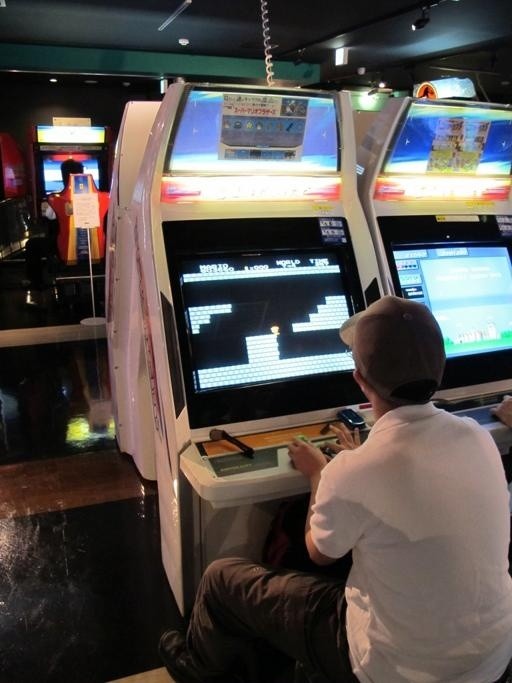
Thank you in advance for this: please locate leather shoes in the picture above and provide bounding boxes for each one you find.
[160,628,203,682]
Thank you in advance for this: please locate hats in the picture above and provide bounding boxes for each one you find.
[339,298,446,402]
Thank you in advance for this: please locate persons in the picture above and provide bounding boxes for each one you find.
[43,157,110,266]
[486,394,512,428]
[155,292,512,683]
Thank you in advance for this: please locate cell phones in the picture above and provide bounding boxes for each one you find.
[336,409,365,430]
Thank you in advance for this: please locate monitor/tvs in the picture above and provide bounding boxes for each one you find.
[376,213,512,392]
[161,214,374,430]
[42,152,101,195]
